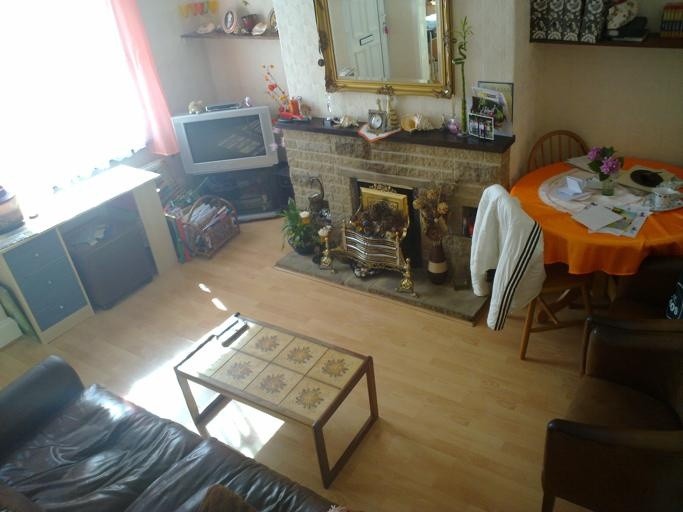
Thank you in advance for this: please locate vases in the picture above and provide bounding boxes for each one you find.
[602,174,615,196]
[426,235,449,285]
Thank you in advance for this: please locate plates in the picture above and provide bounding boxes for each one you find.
[196,10,278,35]
[642,192,682,212]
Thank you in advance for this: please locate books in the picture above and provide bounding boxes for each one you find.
[571,192,651,241]
[180,201,238,254]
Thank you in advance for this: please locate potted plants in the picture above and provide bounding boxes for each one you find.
[241,1,256,32]
[451,15,470,135]
[277,196,317,256]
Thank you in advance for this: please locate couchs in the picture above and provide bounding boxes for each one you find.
[0,356,344,511]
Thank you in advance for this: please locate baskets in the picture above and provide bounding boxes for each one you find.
[165,195,240,258]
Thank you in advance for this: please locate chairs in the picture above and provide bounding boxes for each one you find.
[487,184,592,358]
[539,312,683,511]
[528,129,590,173]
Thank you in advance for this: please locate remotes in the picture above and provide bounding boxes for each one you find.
[219,320,247,345]
[211,317,238,336]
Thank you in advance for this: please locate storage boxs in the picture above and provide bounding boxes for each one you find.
[69,218,154,310]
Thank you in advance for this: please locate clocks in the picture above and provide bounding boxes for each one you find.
[368,109,386,134]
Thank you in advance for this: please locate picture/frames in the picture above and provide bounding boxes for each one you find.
[267,8,278,33]
[223,10,238,33]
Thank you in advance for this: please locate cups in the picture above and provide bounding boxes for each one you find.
[654,188,679,209]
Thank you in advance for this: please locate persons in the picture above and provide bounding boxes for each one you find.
[468,102,504,139]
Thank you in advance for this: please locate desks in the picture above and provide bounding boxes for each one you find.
[0,163,182,346]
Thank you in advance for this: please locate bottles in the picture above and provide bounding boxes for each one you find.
[290,100,299,115]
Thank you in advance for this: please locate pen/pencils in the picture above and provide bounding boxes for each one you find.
[216,320,238,340]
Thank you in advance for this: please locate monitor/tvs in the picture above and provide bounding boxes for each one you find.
[171,106,279,175]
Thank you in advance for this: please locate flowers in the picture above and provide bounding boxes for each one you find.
[259,63,290,112]
[586,145,625,181]
[411,182,450,240]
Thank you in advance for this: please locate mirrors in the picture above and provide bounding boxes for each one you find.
[313,0,454,98]
